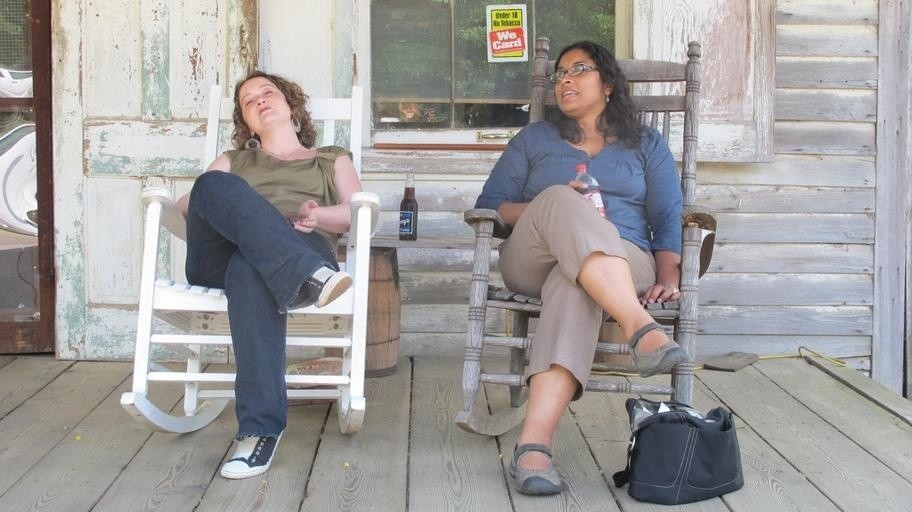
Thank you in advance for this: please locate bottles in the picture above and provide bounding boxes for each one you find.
[398,169,420,240]
[573,163,609,221]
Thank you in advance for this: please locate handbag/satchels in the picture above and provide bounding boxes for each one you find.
[612,392,747,508]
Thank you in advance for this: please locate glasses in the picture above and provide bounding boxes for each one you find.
[549,64,601,84]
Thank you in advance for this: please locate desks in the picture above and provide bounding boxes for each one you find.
[336,211,507,378]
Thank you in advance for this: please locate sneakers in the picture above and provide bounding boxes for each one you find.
[306,267,355,309]
[219,425,284,480]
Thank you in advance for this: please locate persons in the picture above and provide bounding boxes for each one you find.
[173,70,362,480]
[474,42,689,495]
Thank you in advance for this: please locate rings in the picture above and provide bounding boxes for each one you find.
[673,288,680,295]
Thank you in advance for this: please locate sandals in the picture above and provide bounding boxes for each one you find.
[626,320,689,379]
[507,442,564,497]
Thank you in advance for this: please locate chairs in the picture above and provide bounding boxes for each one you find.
[121,83,381,437]
[454,34,719,438]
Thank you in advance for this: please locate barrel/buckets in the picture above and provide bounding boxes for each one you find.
[336,246,404,378]
